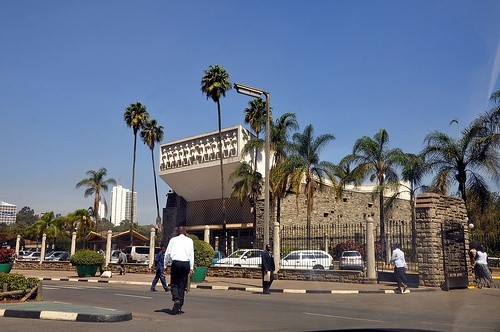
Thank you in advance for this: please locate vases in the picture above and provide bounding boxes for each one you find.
[186,233,216,284]
[0,246,18,274]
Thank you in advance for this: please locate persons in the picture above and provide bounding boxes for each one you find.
[151,246,171,292]
[470,246,476,263]
[475,245,493,287]
[390,243,408,292]
[118,249,127,275]
[262,244,275,293]
[163,227,194,313]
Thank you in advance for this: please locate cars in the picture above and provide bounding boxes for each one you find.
[214,249,263,268]
[339,251,364,269]
[211,251,222,265]
[280,250,334,270]
[17,251,71,261]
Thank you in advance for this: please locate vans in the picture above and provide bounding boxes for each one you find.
[124,246,160,262]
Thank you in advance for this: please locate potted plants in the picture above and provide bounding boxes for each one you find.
[68,247,107,278]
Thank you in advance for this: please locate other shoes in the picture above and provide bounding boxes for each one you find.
[165,288,170,292]
[151,288,157,291]
[395,288,401,290]
[403,285,408,292]
[263,291,271,294]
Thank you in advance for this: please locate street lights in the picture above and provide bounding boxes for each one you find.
[234,83,270,250]
